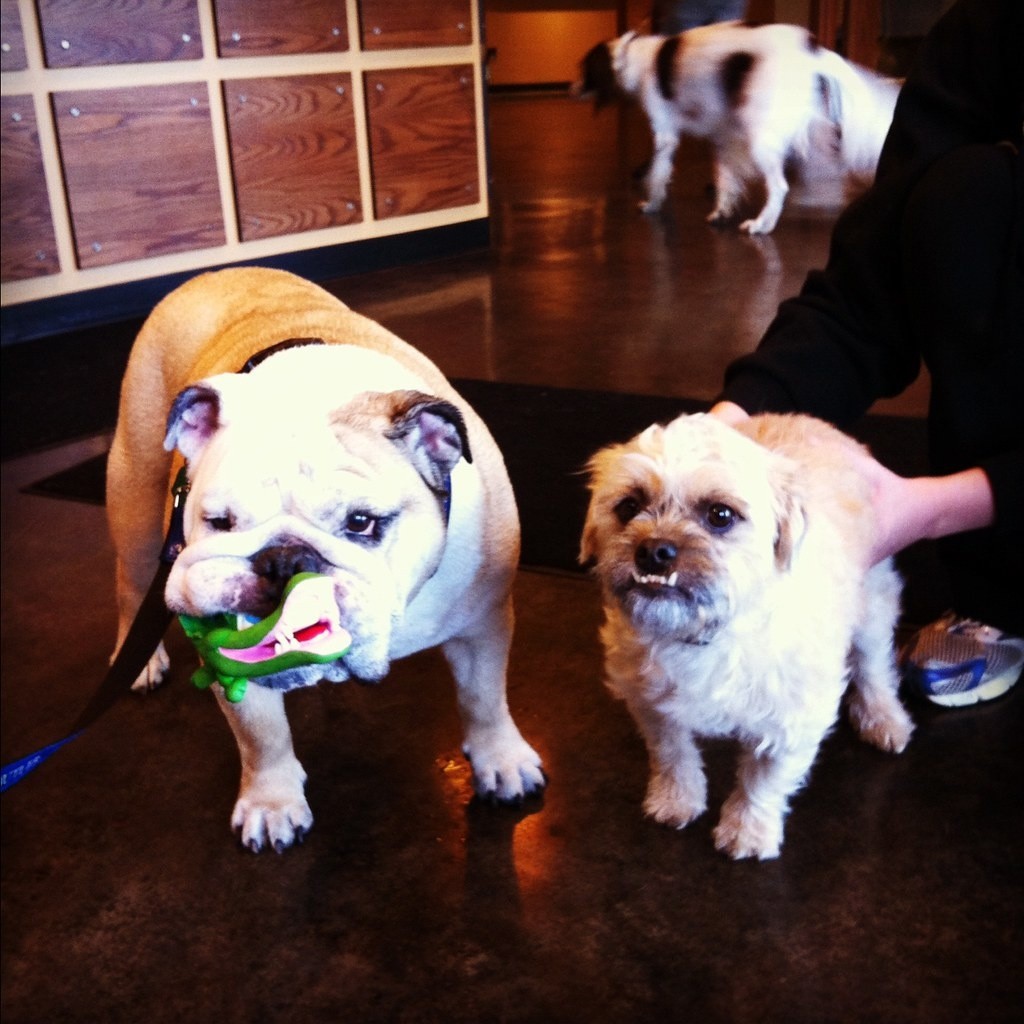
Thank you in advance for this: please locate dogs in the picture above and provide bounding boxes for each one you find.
[106,267,552,865]
[577,22,906,242]
[579,405,918,861]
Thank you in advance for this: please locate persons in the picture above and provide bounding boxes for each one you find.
[711,0,1024,706]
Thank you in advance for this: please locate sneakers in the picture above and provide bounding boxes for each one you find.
[897,613,1024,707]
